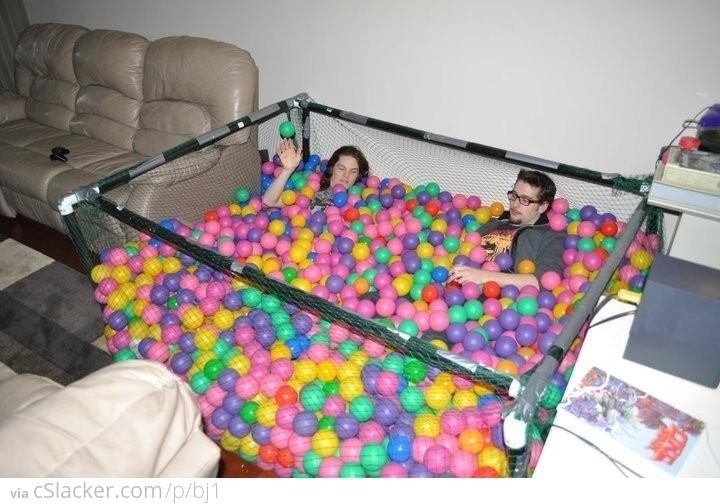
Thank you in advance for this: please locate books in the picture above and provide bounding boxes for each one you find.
[648,162,720,214]
[660,144,719,193]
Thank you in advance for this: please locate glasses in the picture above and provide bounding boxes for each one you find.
[507,190,541,206]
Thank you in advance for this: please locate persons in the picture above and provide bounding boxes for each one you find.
[90,119,663,477]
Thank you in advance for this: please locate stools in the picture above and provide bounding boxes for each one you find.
[0,358,225,477]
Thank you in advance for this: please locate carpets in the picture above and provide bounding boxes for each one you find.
[0,235,117,385]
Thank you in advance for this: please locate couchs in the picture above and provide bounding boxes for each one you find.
[0,22,264,251]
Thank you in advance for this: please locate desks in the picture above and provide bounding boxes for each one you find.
[528,295,720,482]
